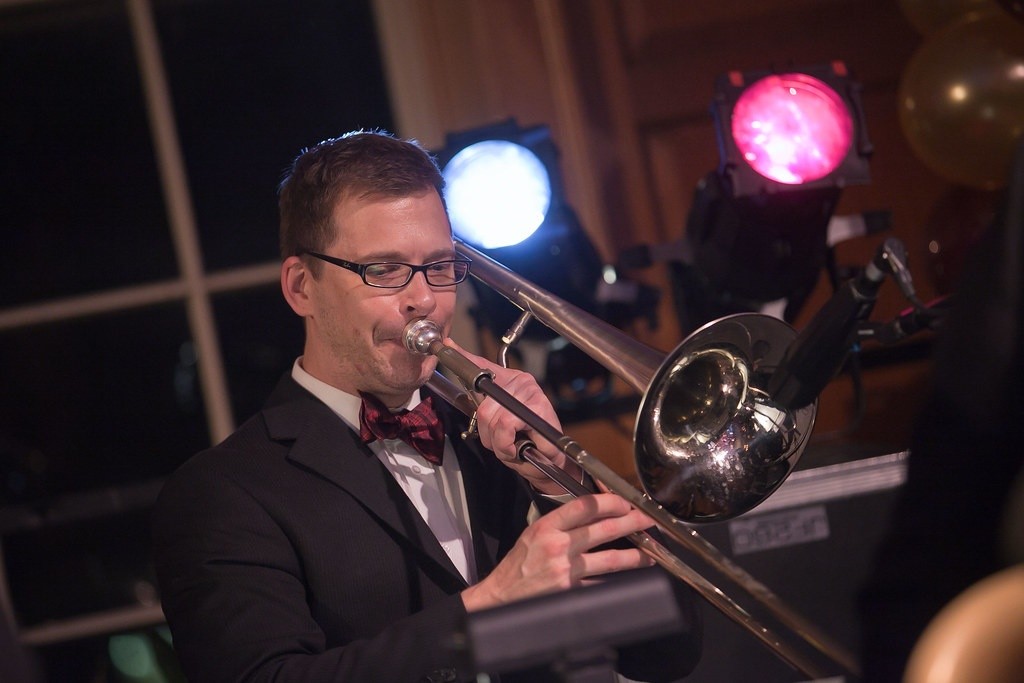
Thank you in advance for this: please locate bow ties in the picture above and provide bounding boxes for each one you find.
[356,388,449,468]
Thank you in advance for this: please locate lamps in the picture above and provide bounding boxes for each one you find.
[669,55,879,344]
[428,118,627,391]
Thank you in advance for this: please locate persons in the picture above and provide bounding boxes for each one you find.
[152,130,704,683]
[902,136,1024,683]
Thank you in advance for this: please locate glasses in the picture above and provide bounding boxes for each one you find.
[303,251,473,287]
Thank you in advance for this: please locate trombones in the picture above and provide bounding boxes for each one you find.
[402,240,1024,683]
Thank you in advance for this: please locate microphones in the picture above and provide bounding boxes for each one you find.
[767,242,902,411]
